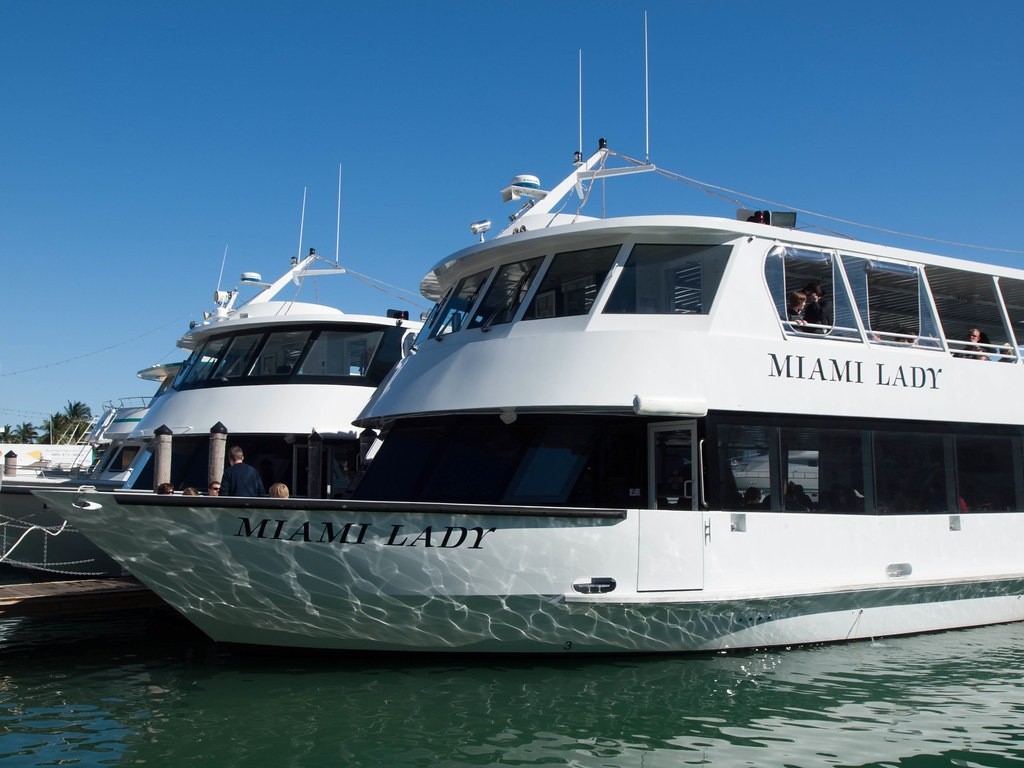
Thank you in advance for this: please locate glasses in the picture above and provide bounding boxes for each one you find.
[211,488,220,491]
[168,491,174,494]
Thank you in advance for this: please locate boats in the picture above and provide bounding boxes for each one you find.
[0,163,454,589]
[32,12,1023,654]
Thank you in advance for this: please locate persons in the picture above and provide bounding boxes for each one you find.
[183,487,198,495]
[268,483,290,498]
[954,327,1016,363]
[207,481,221,496]
[157,483,174,494]
[894,328,917,343]
[744,484,812,511]
[218,446,265,497]
[787,281,833,334]
